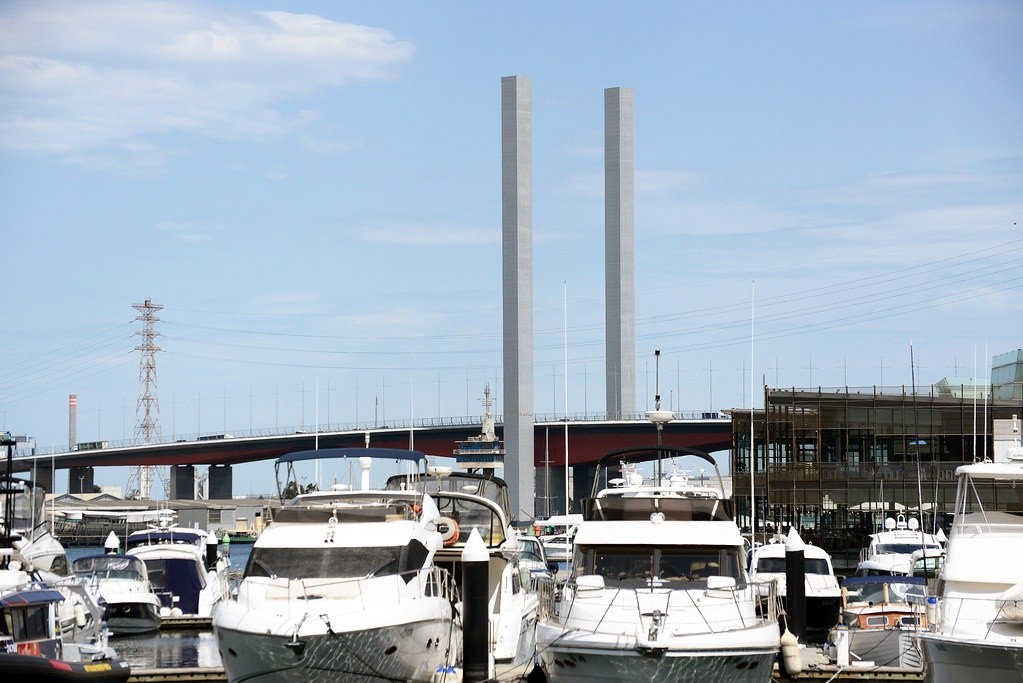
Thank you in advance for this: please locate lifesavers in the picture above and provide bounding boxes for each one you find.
[442,518,460,546]
[426,516,456,541]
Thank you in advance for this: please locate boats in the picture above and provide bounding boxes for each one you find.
[533,514,584,562]
[837,575,930,629]
[72,555,163,634]
[384,470,558,620]
[213,448,497,683]
[854,554,915,578]
[854,516,940,557]
[918,414,1023,683]
[747,542,841,643]
[126,543,231,631]
[0,581,132,683]
[118,503,208,553]
[426,491,547,682]
[908,548,947,580]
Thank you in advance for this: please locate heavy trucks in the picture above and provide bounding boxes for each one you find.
[197,434,235,440]
[74,441,108,451]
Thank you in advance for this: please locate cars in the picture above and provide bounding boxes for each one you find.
[534,420,539,423]
[143,443,151,446]
[352,427,361,430]
[318,430,323,433]
[558,418,570,422]
[172,439,186,443]
[372,426,380,429]
[379,426,389,429]
[296,430,306,434]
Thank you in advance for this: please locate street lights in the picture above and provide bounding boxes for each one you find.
[301,475,308,490]
[77,474,85,494]
[537,349,780,683]
[670,389,673,411]
